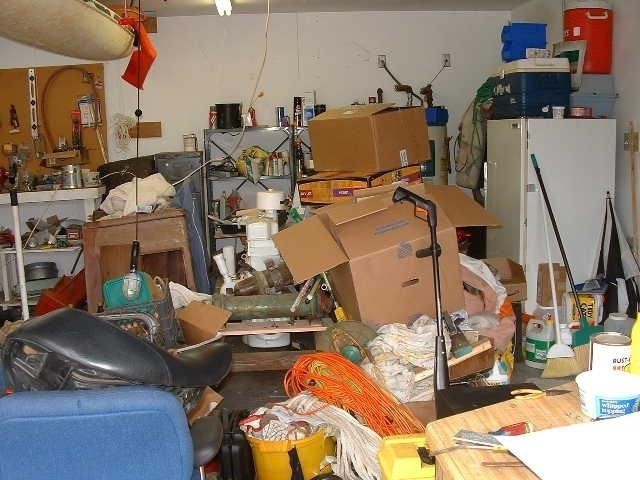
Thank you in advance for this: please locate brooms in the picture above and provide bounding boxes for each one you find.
[541,187,583,379]
[531,152,604,369]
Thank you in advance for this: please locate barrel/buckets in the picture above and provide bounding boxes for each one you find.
[604,312,635,337]
[526,316,554,369]
[533,305,564,323]
[561,293,605,326]
[591,331,633,371]
[558,323,580,346]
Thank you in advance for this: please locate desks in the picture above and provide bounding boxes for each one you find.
[423,380,597,480]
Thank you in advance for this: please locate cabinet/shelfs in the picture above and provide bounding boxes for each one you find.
[2,124,325,374]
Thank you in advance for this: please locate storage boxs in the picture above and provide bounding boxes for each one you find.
[270,56,570,386]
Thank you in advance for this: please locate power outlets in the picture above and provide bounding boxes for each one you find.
[441,53,452,68]
[377,55,388,69]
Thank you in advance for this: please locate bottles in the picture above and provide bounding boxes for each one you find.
[248,107,255,128]
[270,151,289,177]
[210,106,218,129]
[277,107,284,126]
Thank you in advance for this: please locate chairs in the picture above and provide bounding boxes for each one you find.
[1,307,230,480]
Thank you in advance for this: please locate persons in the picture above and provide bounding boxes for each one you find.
[8,104,20,131]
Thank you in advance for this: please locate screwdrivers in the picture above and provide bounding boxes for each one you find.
[427,421,531,460]
